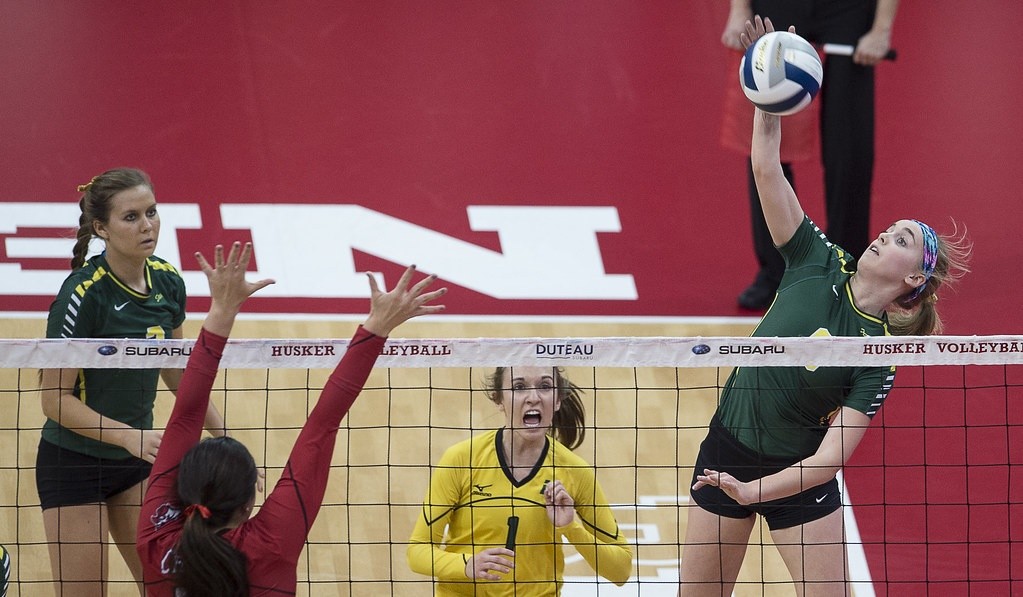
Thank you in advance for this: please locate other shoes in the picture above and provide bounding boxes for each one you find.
[738,277,781,311]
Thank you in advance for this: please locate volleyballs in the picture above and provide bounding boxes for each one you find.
[739,31,823,116]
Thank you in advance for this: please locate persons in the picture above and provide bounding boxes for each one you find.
[407,367,634,597]
[35,167,265,597]
[135,241,449,597]
[676,15,973,597]
[720,0,900,311]
